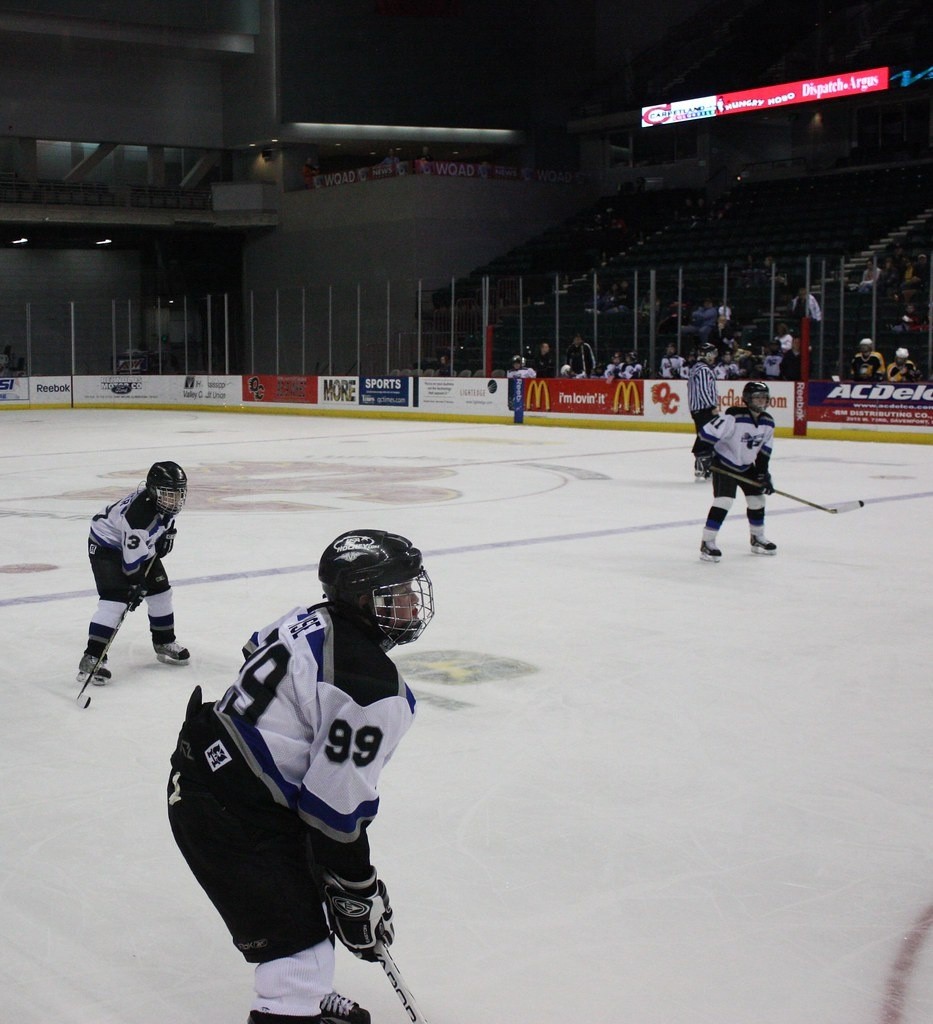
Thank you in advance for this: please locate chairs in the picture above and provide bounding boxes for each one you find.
[0,180,214,210]
[387,369,505,380]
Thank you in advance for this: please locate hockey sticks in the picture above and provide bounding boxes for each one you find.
[374,936,429,1024]
[75,551,158,709]
[708,466,865,514]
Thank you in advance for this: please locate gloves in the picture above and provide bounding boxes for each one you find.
[695,452,717,479]
[756,472,775,495]
[156,528,178,559]
[127,576,150,612]
[322,865,397,962]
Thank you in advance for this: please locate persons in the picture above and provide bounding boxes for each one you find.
[75,460,190,686]
[691,381,778,562]
[166,530,436,1024]
[303,145,933,384]
[686,341,720,478]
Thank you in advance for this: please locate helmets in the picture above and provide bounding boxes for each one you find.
[740,382,770,405]
[316,528,422,607]
[697,343,719,360]
[512,355,523,363]
[896,348,910,358]
[146,461,189,501]
[627,350,639,361]
[859,338,873,348]
[613,352,624,359]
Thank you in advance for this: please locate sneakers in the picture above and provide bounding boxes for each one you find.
[152,640,191,665]
[76,654,113,686]
[750,534,778,555]
[319,991,374,1024]
[699,539,723,562]
